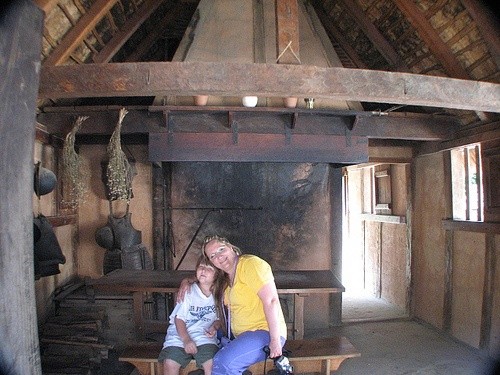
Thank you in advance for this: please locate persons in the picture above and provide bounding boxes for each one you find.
[158,252,222,375]
[201,234,288,375]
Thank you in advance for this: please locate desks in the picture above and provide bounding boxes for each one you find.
[90,269,345,345]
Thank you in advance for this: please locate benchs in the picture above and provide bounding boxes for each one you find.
[119,336,361,375]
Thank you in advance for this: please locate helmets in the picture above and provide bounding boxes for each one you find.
[95,223,117,251]
[34,164,57,200]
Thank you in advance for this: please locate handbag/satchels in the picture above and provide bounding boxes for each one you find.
[221,336,232,347]
